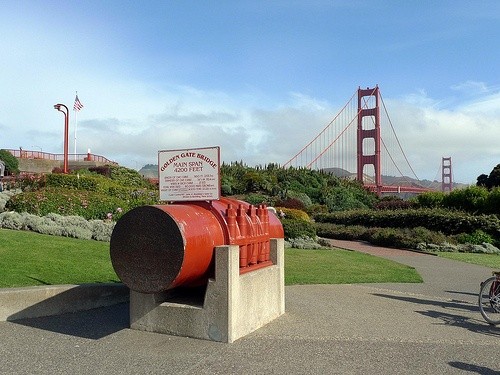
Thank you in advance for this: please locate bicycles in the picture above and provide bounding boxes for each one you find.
[478,271,500,324]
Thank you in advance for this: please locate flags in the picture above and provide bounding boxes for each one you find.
[73,94,83,112]
